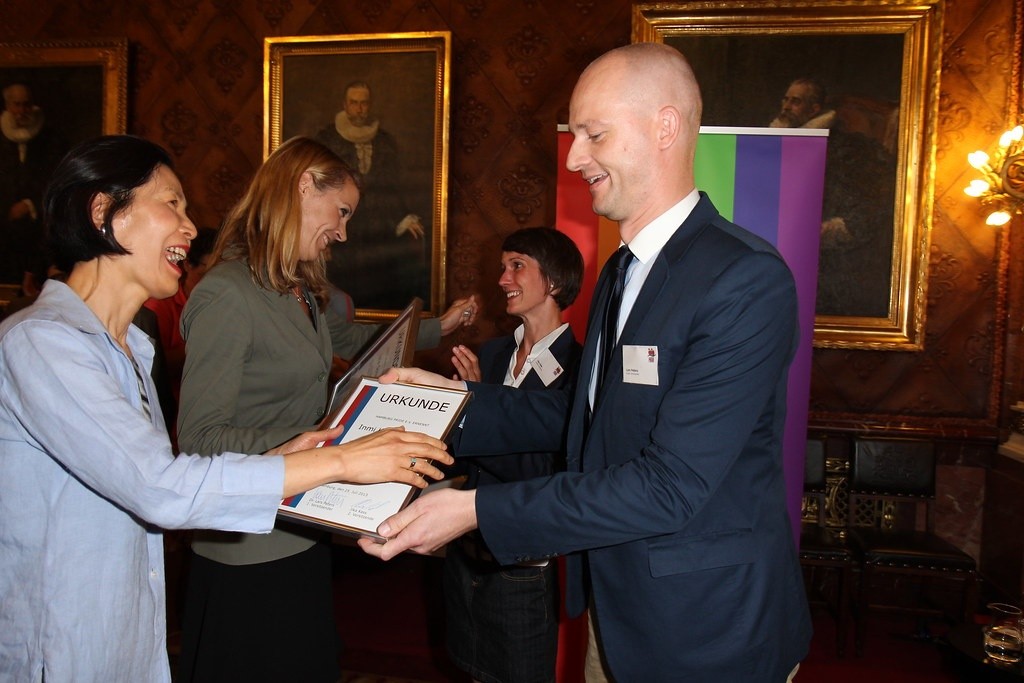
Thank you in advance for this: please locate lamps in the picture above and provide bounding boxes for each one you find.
[966,126,1023,227]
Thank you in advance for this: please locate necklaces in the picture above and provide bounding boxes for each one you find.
[289,287,303,302]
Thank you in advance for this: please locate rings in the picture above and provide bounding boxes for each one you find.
[464,311,470,317]
[409,458,416,470]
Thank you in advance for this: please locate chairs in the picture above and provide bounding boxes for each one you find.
[841,432,979,665]
[791,430,854,657]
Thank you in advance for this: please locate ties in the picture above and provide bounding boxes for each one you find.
[589,246,635,421]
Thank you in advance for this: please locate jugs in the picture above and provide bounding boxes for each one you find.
[981,602,1024,666]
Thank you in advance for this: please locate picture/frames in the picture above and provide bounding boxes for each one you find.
[630,0,950,350]
[0,37,133,311]
[265,29,451,325]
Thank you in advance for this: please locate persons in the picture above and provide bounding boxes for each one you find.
[0,134,455,683]
[357,43,814,683]
[424,227,584,683]
[175,136,479,683]
[0,226,217,550]
